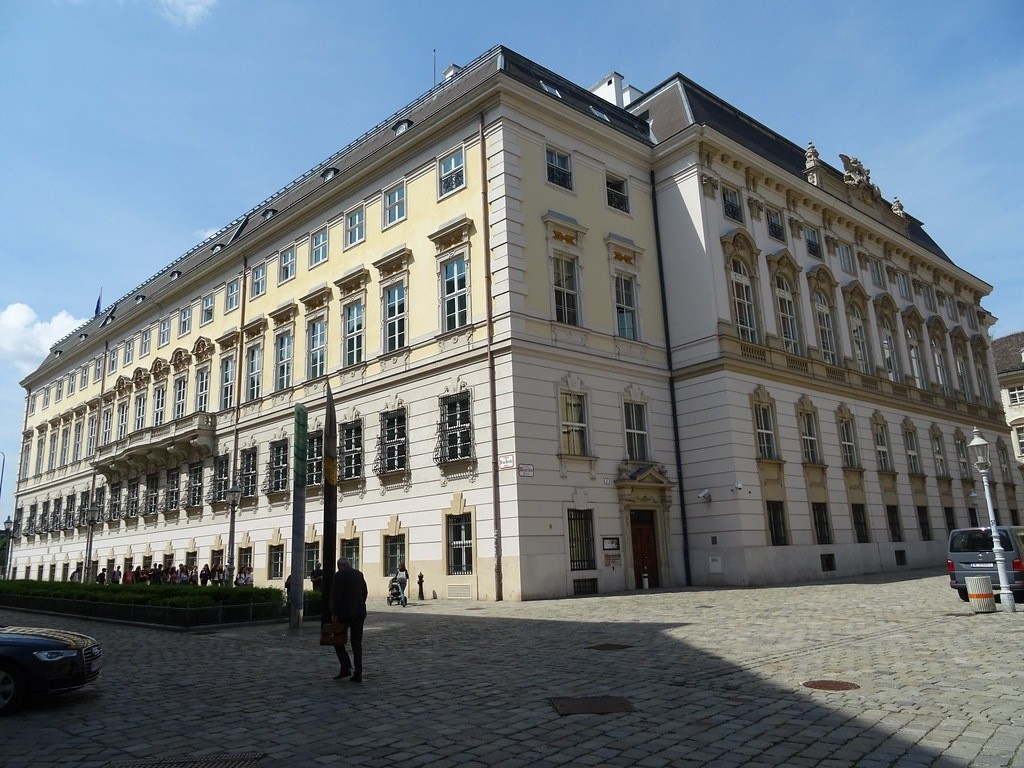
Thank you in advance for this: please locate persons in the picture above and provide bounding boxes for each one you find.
[96,568,107,585]
[329,556,368,682]
[111,566,122,584]
[308,561,323,587]
[392,561,409,607]
[122,561,254,589]
[70,567,82,583]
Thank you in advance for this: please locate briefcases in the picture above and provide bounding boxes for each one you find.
[320,622,348,645]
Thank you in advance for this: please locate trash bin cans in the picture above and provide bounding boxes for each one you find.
[965,575,997,613]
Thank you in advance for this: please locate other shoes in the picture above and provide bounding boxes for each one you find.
[332,667,351,679]
[350,671,363,682]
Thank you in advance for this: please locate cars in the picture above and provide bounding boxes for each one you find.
[0,623,104,713]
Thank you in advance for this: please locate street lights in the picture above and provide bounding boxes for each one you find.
[3,515,15,580]
[85,508,99,586]
[967,425,1016,613]
[223,485,243,589]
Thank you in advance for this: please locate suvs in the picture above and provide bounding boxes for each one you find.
[946,525,1024,603]
